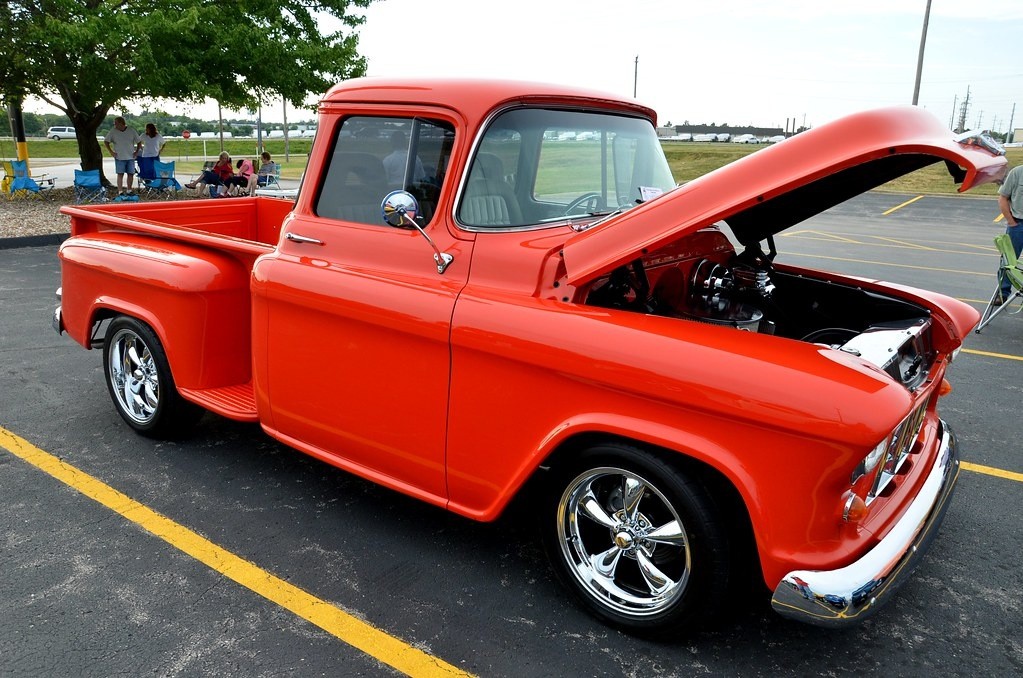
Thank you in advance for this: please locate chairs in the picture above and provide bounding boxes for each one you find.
[3,159,59,207]
[186,158,216,197]
[143,159,182,201]
[73,169,105,205]
[233,160,259,197]
[0,161,31,202]
[322,151,391,226]
[249,163,284,198]
[975,233,1023,334]
[460,152,524,225]
[134,155,160,199]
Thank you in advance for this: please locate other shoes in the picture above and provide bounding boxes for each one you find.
[192,193,204,198]
[184,183,196,189]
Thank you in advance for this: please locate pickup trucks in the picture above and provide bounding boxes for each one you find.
[51,76,1011,639]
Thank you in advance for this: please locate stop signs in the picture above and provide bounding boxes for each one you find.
[183,130,190,139]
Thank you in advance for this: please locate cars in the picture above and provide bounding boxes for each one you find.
[508,126,788,143]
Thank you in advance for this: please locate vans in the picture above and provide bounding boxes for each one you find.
[47,126,77,141]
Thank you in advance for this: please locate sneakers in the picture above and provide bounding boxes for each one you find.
[991,296,1007,307]
[119,193,124,196]
[127,192,135,196]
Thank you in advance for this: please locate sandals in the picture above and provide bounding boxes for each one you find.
[239,191,250,197]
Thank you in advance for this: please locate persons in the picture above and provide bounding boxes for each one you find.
[383,131,427,185]
[993,164,1023,305]
[104,117,141,195]
[184,151,233,197]
[216,159,254,198]
[139,124,165,191]
[239,152,276,197]
